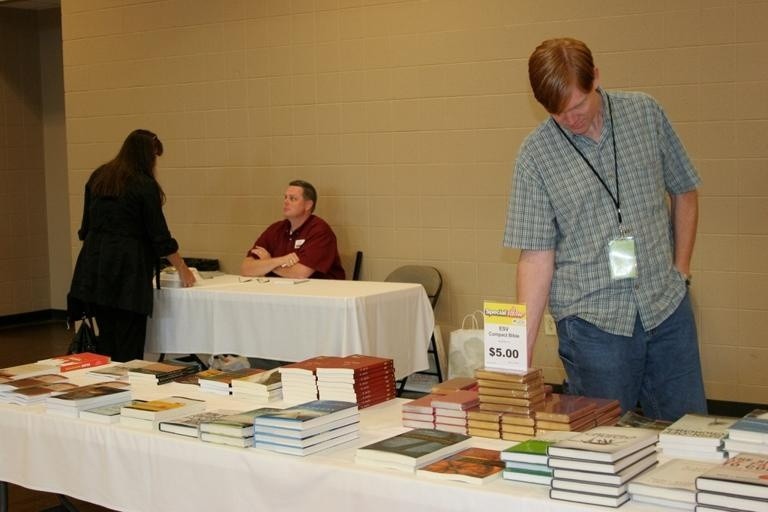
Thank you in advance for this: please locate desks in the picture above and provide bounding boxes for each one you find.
[144,272,436,382]
[0,357,700,511]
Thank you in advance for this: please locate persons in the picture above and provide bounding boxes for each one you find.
[66,130,197,363]
[240,180,345,370]
[504,38,709,423]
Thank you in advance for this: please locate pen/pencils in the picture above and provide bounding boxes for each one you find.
[293,280,310,284]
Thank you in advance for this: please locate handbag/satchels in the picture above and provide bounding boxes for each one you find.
[65,323,109,356]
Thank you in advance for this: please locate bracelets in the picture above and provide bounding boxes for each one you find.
[684,272,692,286]
[174,257,184,269]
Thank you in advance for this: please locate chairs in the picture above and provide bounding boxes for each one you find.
[383,266,445,396]
[339,249,365,282]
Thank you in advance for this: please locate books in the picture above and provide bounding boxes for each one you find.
[356,366,768,512]
[0,353,397,457]
